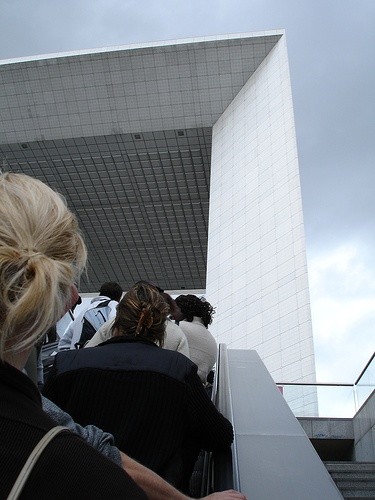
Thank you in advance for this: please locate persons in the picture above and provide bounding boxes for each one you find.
[0,172,246,500]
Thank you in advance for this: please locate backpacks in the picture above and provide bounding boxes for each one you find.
[74,301,112,350]
